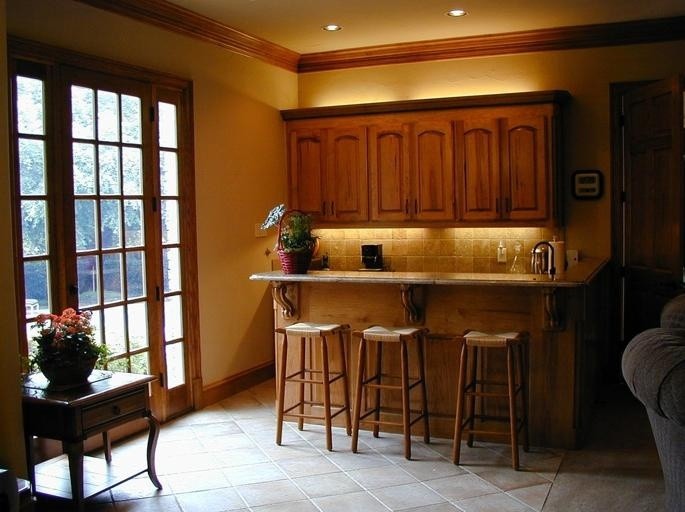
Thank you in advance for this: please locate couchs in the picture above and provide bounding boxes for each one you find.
[621,293,684,512]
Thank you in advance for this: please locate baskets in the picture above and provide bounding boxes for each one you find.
[276,211,314,274]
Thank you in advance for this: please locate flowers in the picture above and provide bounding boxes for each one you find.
[279,212,322,256]
[17,306,130,379]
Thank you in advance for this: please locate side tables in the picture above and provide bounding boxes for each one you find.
[21,367,163,511]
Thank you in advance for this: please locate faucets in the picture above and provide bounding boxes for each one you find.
[533,241,557,280]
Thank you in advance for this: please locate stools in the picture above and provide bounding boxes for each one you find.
[350,324,430,460]
[274,322,352,452]
[452,328,532,470]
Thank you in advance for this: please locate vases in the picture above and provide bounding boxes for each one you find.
[276,249,313,274]
[35,357,102,394]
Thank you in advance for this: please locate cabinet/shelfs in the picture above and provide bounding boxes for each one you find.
[369,112,459,226]
[457,102,563,227]
[284,114,370,228]
